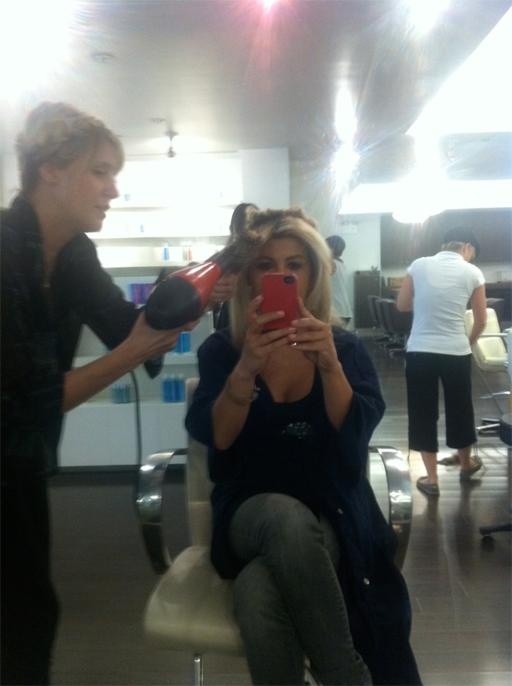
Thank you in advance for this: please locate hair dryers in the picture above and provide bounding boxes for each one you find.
[147,246,237,375]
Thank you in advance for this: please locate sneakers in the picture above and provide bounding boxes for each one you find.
[418,477,439,496]
[460,456,482,478]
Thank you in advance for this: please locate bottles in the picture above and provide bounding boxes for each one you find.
[112,376,134,403]
[164,243,170,259]
[162,372,187,404]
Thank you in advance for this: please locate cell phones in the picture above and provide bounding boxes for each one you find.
[261,272,299,331]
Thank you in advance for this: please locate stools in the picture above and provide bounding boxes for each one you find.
[478,413,512,548]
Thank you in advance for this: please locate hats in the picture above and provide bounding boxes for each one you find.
[441,225,479,249]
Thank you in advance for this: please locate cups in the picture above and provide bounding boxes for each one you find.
[174,331,191,353]
[130,283,155,305]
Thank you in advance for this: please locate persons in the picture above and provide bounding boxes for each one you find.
[184,211,386,686]
[323,235,353,327]
[396,226,488,496]
[0,103,240,683]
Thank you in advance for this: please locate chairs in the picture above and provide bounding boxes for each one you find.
[465,307,512,434]
[134,378,414,685]
[368,293,504,368]
